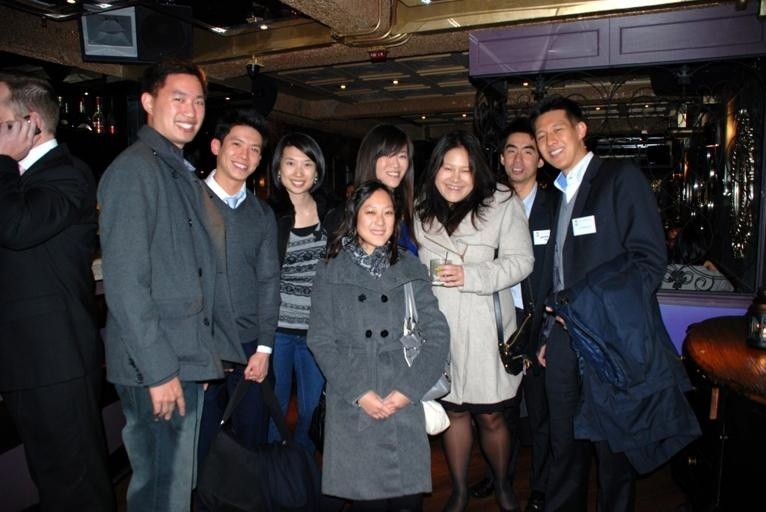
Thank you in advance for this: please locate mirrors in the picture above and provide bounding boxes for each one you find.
[730,109,756,261]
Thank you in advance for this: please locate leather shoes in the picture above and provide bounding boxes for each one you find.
[472,471,513,496]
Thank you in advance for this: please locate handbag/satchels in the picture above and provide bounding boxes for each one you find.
[399,281,452,402]
[493,246,538,376]
[195,368,293,512]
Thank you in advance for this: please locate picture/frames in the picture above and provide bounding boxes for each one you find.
[80,5,144,65]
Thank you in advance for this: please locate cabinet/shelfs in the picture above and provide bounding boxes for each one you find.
[469,5,766,77]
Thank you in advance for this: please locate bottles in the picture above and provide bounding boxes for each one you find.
[76,97,91,128]
[92,96,105,134]
[58,96,63,110]
[64,102,72,124]
[106,98,118,134]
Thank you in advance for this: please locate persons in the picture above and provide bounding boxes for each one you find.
[470,122,560,512]
[355,123,419,260]
[0,70,117,512]
[198,112,282,512]
[414,128,535,511]
[306,180,450,512]
[264,132,328,477]
[530,94,668,512]
[98,58,246,511]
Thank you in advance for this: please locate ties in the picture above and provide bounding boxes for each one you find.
[225,192,244,209]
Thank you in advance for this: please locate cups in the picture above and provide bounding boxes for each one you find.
[430,258,451,282]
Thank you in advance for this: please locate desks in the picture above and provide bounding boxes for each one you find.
[678,314,766,429]
